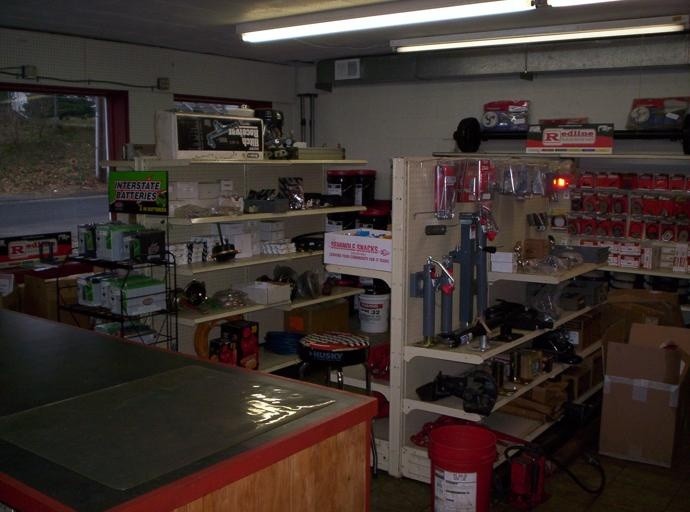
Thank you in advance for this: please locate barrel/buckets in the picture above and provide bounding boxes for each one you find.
[427,424,498,512]
[326,168,389,230]
[329,272,374,286]
[355,284,391,334]
